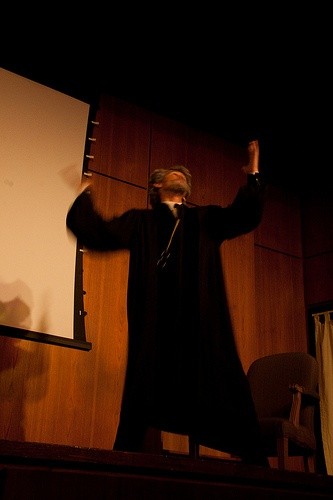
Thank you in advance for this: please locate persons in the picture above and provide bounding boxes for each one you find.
[68,137,272,472]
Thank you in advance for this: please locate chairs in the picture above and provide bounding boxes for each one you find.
[188,351,319,470]
